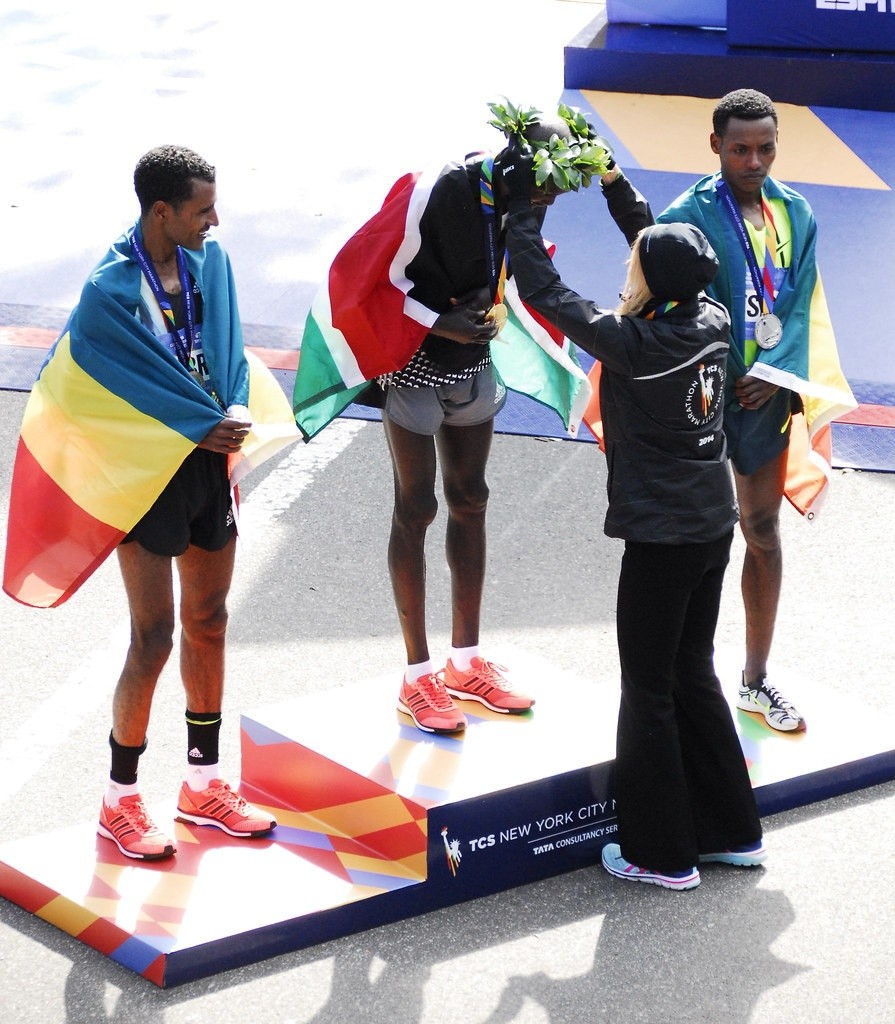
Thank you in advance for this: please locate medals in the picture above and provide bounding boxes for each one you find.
[755,314,783,349]
[189,372,206,390]
[484,304,508,335]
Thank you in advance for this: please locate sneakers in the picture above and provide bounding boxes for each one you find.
[441,658,537,715]
[700,841,767,866]
[175,779,278,838]
[97,793,178,860]
[400,674,469,735]
[603,842,701,891]
[736,669,808,732]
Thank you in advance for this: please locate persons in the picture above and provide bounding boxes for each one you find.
[295,112,593,736]
[489,115,771,891]
[654,86,860,734]
[2,146,277,858]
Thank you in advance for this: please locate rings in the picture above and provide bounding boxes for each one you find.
[232,430,236,440]
